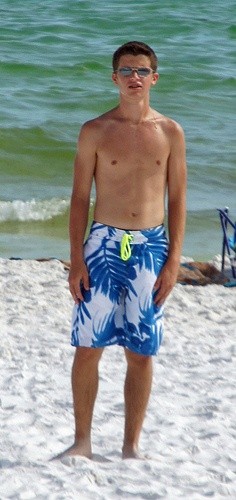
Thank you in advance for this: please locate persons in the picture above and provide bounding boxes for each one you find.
[49,41,187,462]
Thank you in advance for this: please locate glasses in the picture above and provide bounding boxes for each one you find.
[113,67,156,79]
